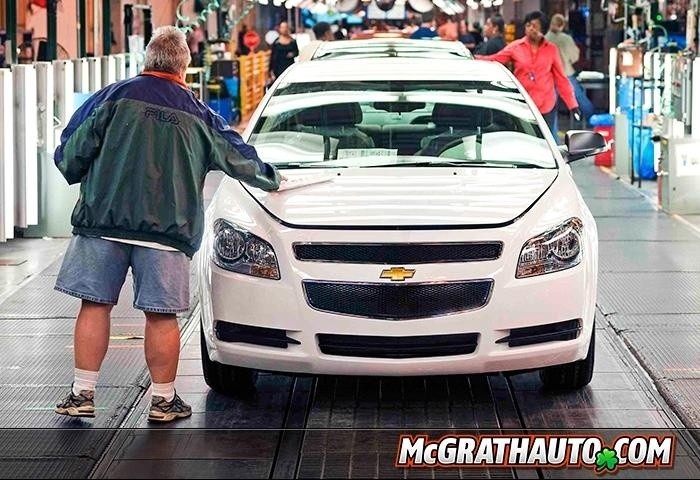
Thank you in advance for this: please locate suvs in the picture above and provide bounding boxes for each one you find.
[192,47,605,397]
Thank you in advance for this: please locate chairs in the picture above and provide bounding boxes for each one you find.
[278,93,373,161]
[421,97,506,162]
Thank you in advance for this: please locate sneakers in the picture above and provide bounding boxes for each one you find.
[148,388,193,423]
[54,380,97,418]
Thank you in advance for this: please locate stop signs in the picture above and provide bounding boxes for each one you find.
[244,31,262,50]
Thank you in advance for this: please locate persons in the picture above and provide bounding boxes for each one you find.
[54,24,290,424]
[237,9,595,144]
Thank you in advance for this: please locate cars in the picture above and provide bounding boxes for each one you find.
[655,21,686,53]
[303,33,478,63]
